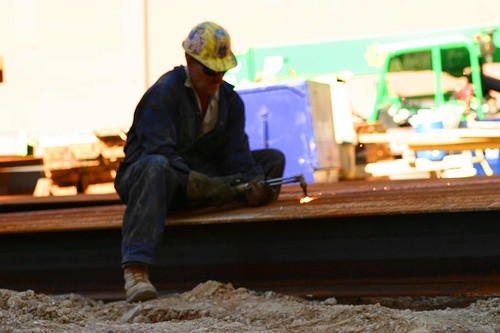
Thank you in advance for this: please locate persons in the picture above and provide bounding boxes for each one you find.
[112,22,287,307]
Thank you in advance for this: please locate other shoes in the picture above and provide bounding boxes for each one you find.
[123,263,158,304]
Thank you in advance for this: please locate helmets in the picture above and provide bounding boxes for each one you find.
[182,21,238,73]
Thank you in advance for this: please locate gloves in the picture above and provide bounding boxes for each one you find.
[186,167,263,199]
[248,178,277,206]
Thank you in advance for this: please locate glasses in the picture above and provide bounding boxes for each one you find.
[195,59,227,77]
